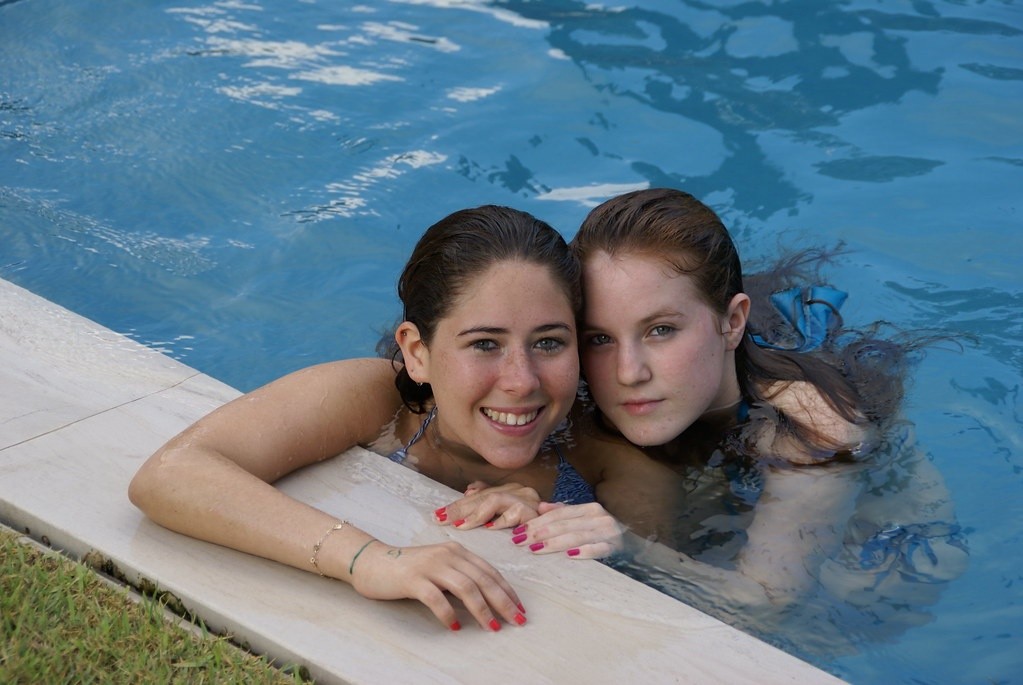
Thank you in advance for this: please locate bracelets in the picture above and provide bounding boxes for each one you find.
[310,521,353,578]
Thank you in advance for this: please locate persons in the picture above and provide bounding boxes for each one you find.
[128,205,588,632]
[512,187,865,559]
